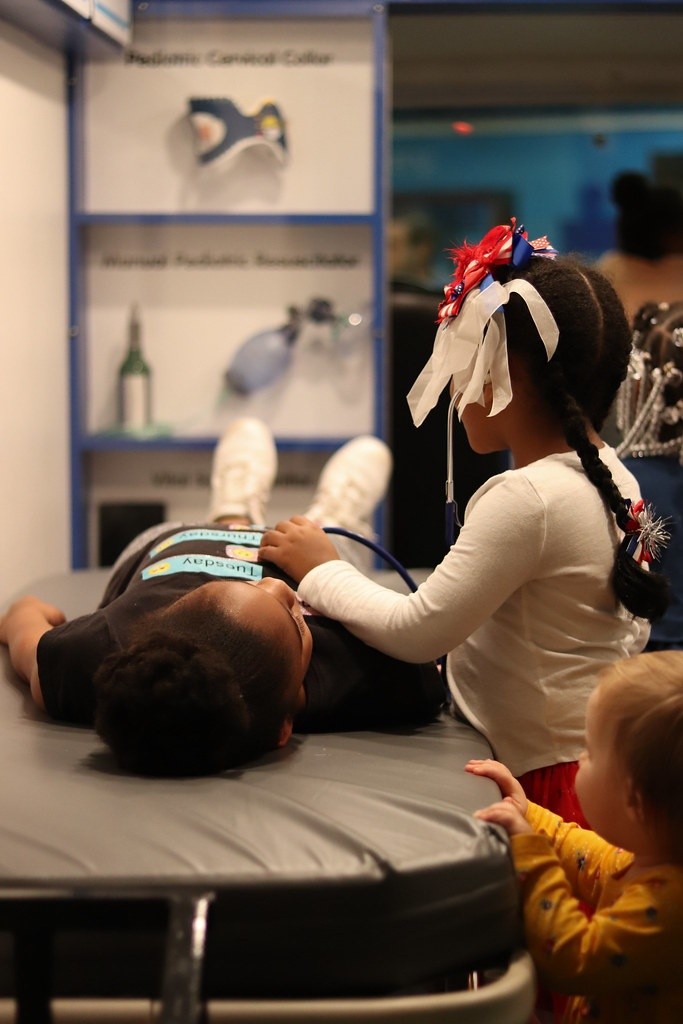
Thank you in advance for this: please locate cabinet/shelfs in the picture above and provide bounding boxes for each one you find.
[62,4,395,573]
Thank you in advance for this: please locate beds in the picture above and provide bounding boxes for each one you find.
[0,560,546,1024]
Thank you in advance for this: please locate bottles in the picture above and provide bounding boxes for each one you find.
[117,307,151,434]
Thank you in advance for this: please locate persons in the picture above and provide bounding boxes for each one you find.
[616,298,682,651]
[463,644,682,1023]
[0,415,449,778]
[257,214,669,1023]
[589,171,683,450]
